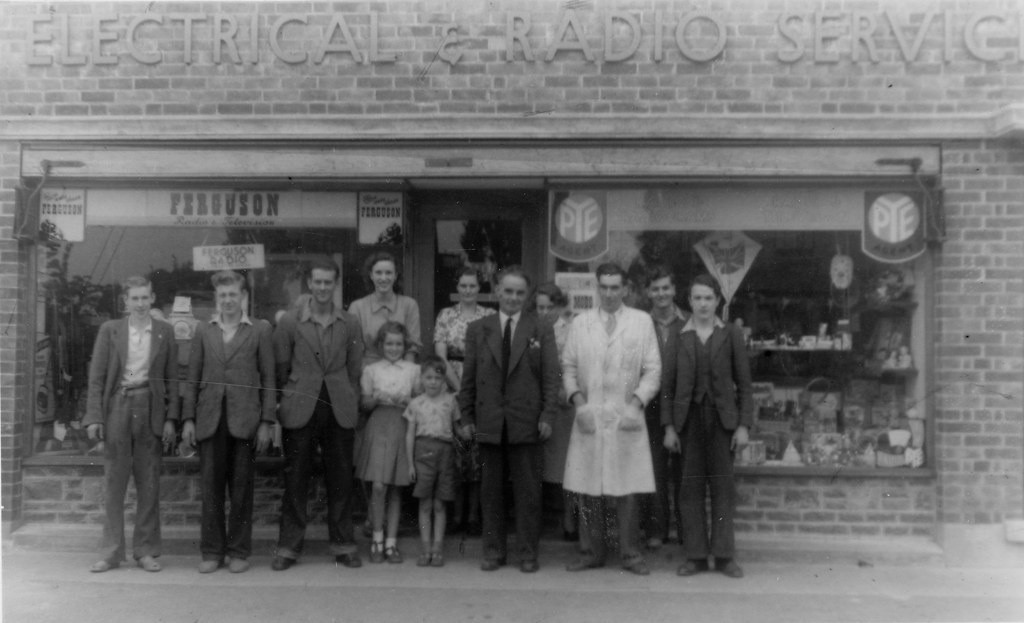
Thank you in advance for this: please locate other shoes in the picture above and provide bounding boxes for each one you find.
[271,556,298,570]
[627,561,652,576]
[430,550,442,566]
[481,557,508,570]
[199,559,224,573]
[335,554,362,567]
[518,560,541,573]
[677,559,709,576]
[715,557,744,579]
[137,555,162,572]
[566,550,607,571]
[369,540,385,562]
[229,557,251,573]
[89,558,121,573]
[416,549,431,566]
[385,545,402,563]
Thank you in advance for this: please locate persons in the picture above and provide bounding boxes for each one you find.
[661,274,754,578]
[349,253,421,529]
[434,266,500,539]
[359,322,423,563]
[181,270,277,574]
[624,274,692,548]
[561,264,663,575]
[271,258,361,570]
[527,281,580,542]
[81,276,180,571]
[403,355,471,567]
[461,265,559,573]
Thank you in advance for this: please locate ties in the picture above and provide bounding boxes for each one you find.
[502,318,513,368]
[606,316,616,337]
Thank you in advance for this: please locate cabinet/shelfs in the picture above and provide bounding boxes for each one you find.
[731,284,921,468]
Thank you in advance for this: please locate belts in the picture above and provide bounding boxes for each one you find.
[115,388,152,396]
